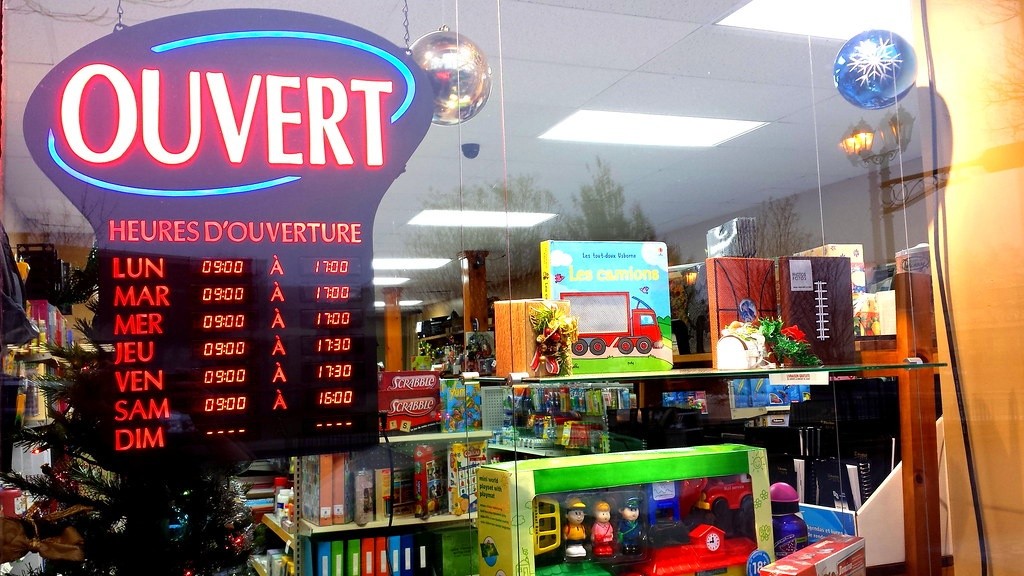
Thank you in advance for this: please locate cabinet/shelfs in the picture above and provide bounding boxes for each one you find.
[449,245,940,576]
[250,432,495,576]
[417,330,464,374]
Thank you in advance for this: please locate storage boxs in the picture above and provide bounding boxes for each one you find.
[379,370,440,432]
[439,379,481,432]
[25,299,74,350]
[540,241,675,375]
[300,439,489,526]
[496,300,573,379]
[761,535,867,576]
[301,526,478,576]
[475,442,776,576]
[667,245,898,370]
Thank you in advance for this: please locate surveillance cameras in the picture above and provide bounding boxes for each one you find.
[461,144,480,159]
